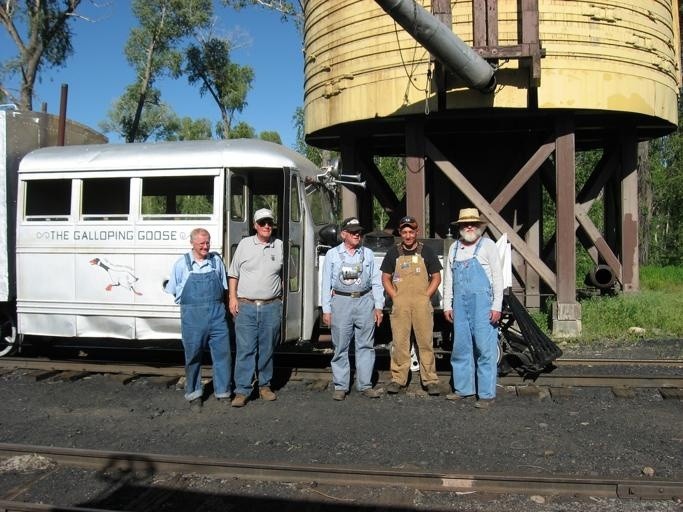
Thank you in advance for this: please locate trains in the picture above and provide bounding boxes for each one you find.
[0,100,564,378]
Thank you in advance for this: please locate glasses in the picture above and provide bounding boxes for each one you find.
[259,221,272,226]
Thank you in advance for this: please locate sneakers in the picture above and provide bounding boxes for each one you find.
[332,383,399,401]
[426,383,495,408]
[257,387,276,401]
[190,387,248,413]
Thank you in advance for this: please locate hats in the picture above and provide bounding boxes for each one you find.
[451,208,488,225]
[399,216,416,228]
[254,208,273,223]
[340,217,364,231]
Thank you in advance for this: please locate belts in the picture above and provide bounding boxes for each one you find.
[332,287,372,298]
[240,298,278,306]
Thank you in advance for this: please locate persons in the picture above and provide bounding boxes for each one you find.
[379,216,442,395]
[443,207,504,408]
[321,217,385,400]
[165,228,233,412]
[227,208,284,407]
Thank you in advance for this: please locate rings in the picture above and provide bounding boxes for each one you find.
[381,314,384,317]
